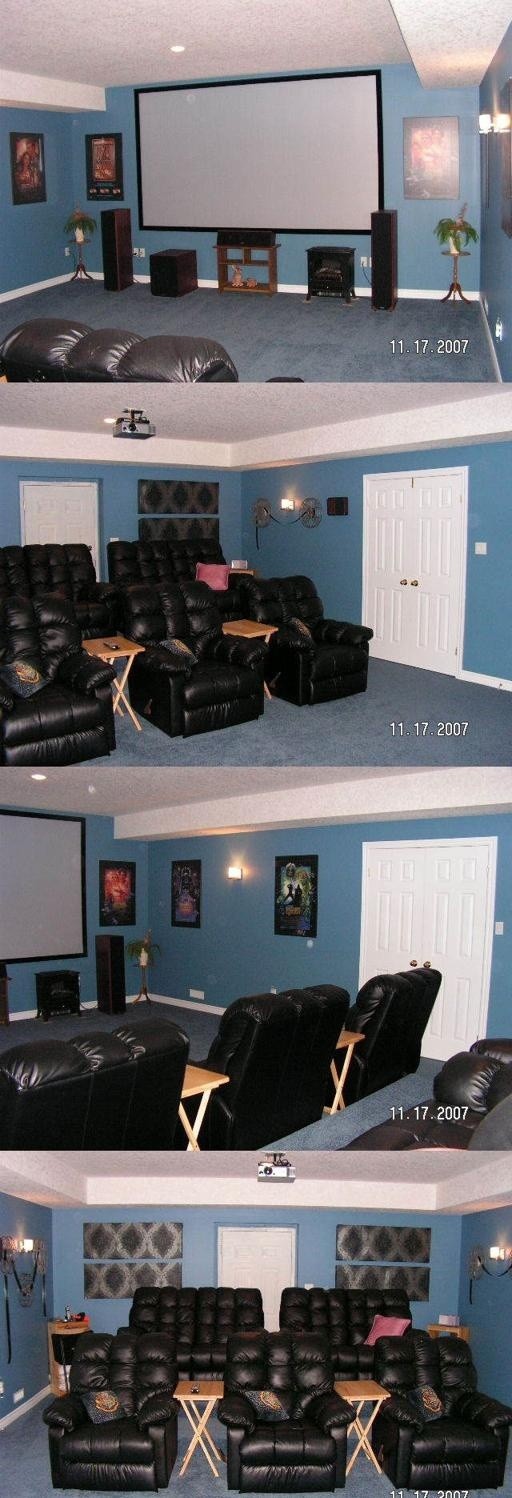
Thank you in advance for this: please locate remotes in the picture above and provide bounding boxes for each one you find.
[191,1383,200,1393]
[103,640,118,649]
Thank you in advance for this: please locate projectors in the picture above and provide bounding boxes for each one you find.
[112,423,156,440]
[257,1162,296,1183]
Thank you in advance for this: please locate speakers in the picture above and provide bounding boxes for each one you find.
[95,935,127,1015]
[217,230,276,247]
[371,210,398,311]
[149,248,198,298]
[100,207,133,292]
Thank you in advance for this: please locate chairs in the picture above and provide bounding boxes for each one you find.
[1,314,305,382]
[0,572,373,765]
[0,967,444,1149]
[42,1330,512,1492]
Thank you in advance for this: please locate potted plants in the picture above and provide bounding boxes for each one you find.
[126,939,162,964]
[63,213,97,241]
[433,216,479,253]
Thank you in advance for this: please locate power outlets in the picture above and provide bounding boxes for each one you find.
[64,248,70,256]
[361,256,368,267]
[133,248,139,256]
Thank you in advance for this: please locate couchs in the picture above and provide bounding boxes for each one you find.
[337,1034,511,1150]
[0,536,232,593]
[116,1286,419,1379]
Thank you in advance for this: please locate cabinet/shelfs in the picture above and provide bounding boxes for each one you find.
[213,244,282,295]
[306,245,357,302]
[48,1318,92,1398]
[34,969,82,1021]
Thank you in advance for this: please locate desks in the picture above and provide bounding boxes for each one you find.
[132,961,154,1007]
[440,250,472,305]
[231,568,255,580]
[427,1324,471,1347]
[68,239,93,281]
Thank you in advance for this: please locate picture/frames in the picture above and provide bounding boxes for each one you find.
[140,248,145,257]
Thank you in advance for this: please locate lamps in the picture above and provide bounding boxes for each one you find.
[488,1243,506,1262]
[280,497,296,511]
[19,1236,34,1252]
[478,111,496,134]
[225,864,243,879]
